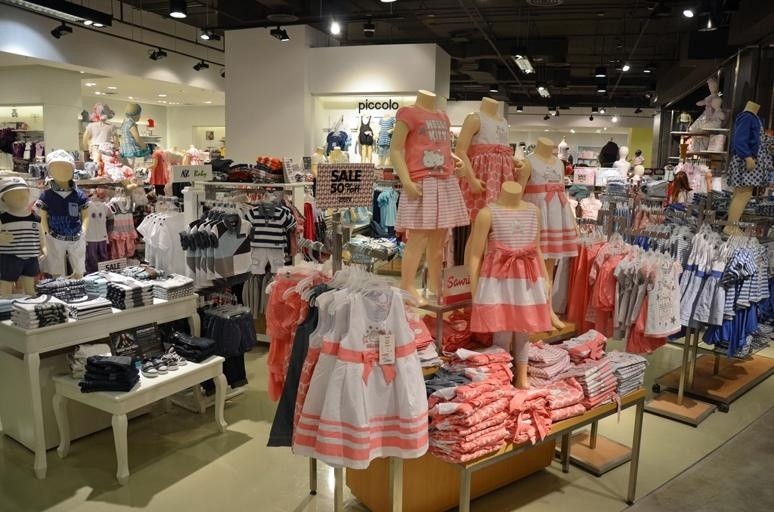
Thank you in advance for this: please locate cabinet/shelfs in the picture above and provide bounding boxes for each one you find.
[574,144,604,168]
[77,114,163,163]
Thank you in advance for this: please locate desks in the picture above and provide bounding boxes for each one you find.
[0,280,210,483]
[316,316,584,512]
[383,384,654,512]
[46,342,232,488]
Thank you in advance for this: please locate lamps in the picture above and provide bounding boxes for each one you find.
[0,1,740,127]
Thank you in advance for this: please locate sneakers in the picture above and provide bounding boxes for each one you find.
[141,350,188,378]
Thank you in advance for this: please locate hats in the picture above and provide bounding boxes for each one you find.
[87,102,116,122]
[0,176,35,200]
[45,149,77,171]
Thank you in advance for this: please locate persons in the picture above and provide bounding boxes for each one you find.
[312,114,395,197]
[390,78,773,388]
[1,102,203,299]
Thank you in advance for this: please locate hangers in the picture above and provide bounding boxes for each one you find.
[678,153,710,169]
[261,237,422,316]
[81,172,315,219]
[574,195,774,279]
